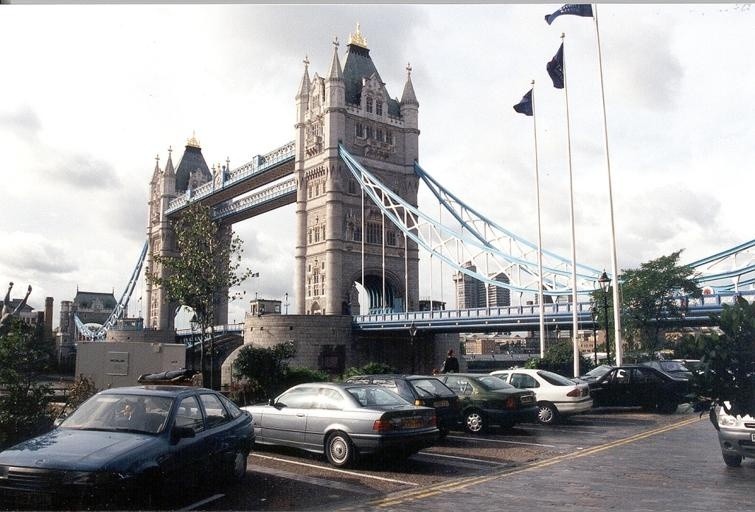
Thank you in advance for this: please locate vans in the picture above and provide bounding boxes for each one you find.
[717,371,755,467]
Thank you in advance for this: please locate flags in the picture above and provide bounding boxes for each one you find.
[546,42,564,89]
[544,4,593,26]
[513,88,533,117]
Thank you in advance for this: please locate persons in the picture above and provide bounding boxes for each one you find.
[554,295,560,313]
[432,369,440,374]
[442,349,461,373]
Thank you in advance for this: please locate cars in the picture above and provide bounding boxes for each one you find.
[488,369,594,426]
[239,381,441,468]
[0,385,256,512]
[573,363,690,414]
[435,373,540,434]
[347,374,464,437]
[644,351,713,387]
